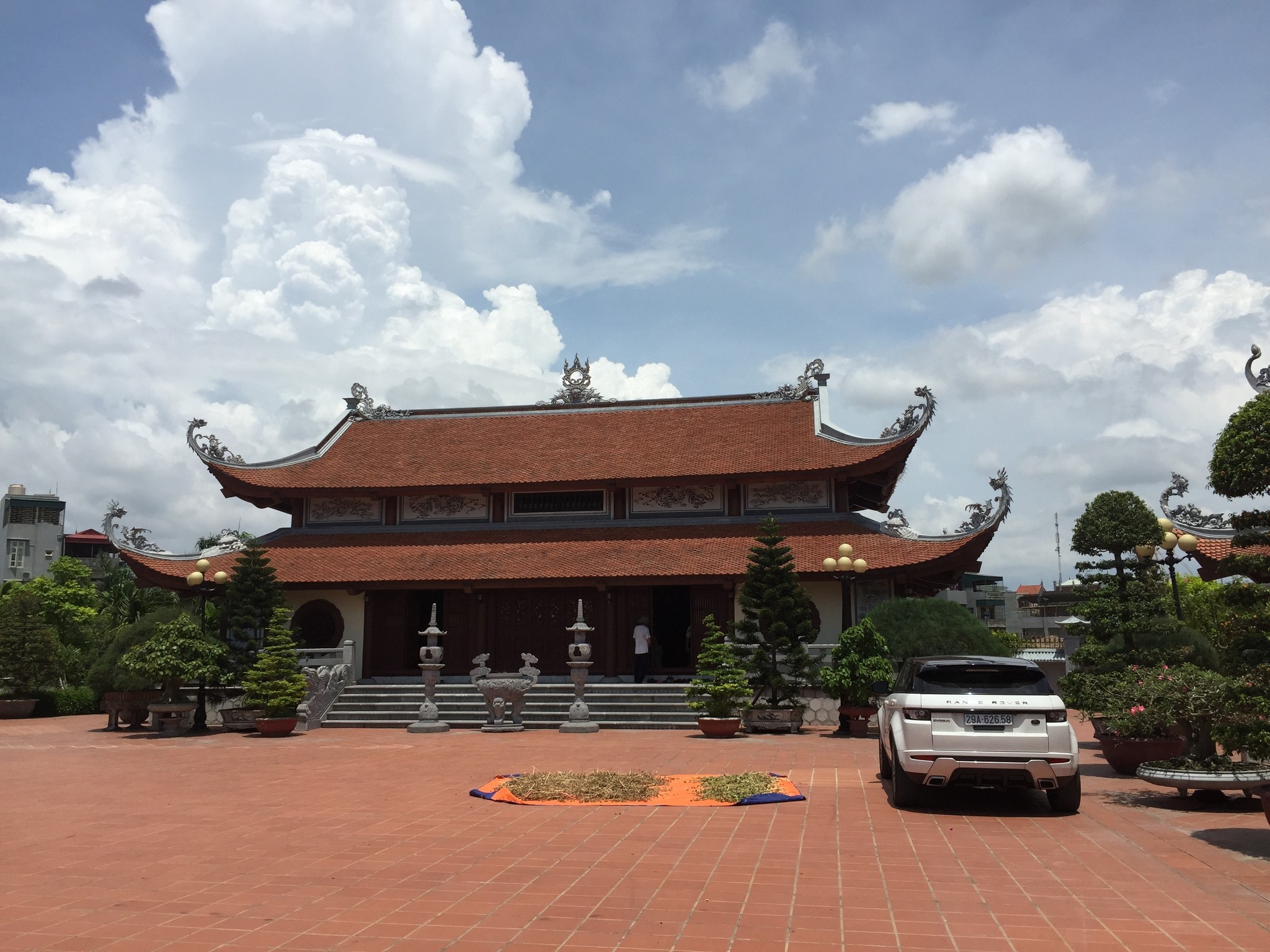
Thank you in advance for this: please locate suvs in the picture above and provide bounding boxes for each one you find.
[869,654,1081,813]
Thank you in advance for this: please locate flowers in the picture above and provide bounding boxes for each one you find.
[1072,660,1270,763]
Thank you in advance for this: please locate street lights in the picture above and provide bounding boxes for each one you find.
[1135,518,1198,621]
[186,559,227,735]
[822,542,867,635]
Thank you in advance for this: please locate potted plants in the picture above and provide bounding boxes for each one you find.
[206,535,294,730]
[724,510,828,733]
[821,617,896,738]
[0,588,66,719]
[683,613,754,738]
[84,605,235,738]
[241,607,310,737]
[1136,752,1270,799]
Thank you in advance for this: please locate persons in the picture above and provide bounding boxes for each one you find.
[653,630,663,667]
[686,625,691,651]
[632,617,651,683]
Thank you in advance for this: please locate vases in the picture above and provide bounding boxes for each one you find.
[1249,783,1270,826]
[1093,732,1189,775]
[1087,716,1113,733]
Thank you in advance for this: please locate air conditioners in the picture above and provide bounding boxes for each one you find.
[22,571,33,579]
